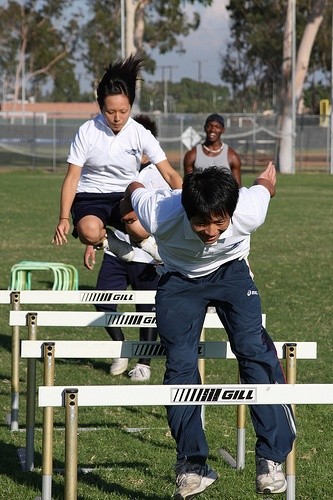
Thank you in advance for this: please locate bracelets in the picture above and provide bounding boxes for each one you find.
[60,218,68,219]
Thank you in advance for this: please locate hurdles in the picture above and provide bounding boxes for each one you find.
[7,308,268,474]
[18,337,319,500]
[0,288,208,434]
[34,382,333,500]
[8,260,82,291]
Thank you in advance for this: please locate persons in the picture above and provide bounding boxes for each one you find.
[119,160,297,500]
[94,114,171,382]
[51,54,183,262]
[183,113,242,188]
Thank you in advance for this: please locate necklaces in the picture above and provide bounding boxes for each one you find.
[203,145,222,153]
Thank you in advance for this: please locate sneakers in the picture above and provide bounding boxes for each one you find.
[93,228,135,262]
[110,357,129,376]
[255,456,288,495]
[130,363,151,382]
[174,461,218,500]
[129,234,163,264]
